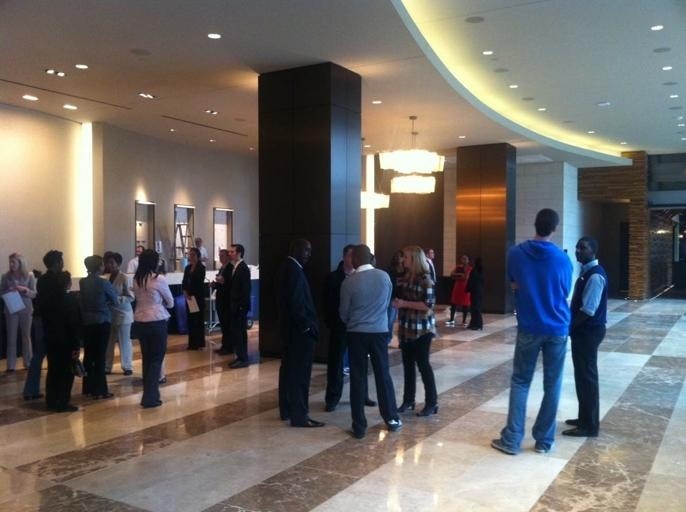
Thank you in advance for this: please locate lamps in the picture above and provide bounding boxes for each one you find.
[378,117,446,173]
[389,174,436,196]
[357,190,389,209]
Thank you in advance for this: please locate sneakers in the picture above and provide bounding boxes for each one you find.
[490,438,518,454]
[536,442,550,453]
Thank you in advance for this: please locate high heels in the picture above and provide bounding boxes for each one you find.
[472,324,482,329]
[417,403,439,416]
[467,325,472,328]
[398,399,416,412]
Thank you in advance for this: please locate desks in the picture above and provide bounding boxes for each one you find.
[0,264,259,336]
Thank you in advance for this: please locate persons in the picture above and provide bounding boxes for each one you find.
[194,237,209,268]
[491,208,573,457]
[227,244,251,368]
[182,247,206,351]
[272,235,441,439]
[560,237,609,437]
[464,254,485,330]
[208,249,234,356]
[445,254,473,326]
[0,253,38,373]
[20,244,175,412]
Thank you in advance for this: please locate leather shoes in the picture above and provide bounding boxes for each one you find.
[388,419,402,431]
[325,403,337,412]
[364,399,377,406]
[445,320,455,325]
[214,348,232,355]
[303,418,324,427]
[229,359,249,369]
[563,428,580,435]
[24,370,166,413]
[566,420,578,425]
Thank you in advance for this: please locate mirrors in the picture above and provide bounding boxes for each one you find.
[213,208,234,270]
[135,201,155,257]
[174,205,195,259]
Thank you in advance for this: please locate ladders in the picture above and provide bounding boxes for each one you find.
[177,222,201,258]
